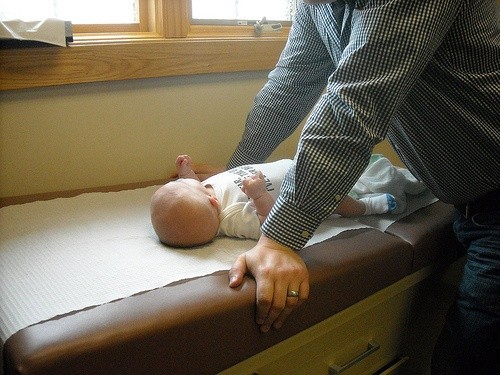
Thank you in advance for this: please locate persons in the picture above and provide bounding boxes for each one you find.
[168,0,500,375]
[150,154,397,247]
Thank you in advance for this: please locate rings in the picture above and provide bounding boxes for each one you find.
[286,290,299,297]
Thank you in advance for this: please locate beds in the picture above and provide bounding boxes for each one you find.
[0,177,465,375]
